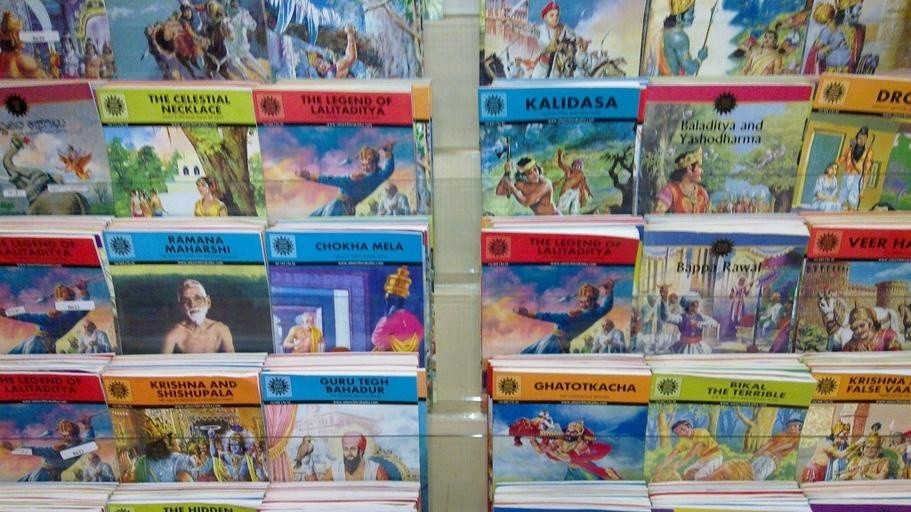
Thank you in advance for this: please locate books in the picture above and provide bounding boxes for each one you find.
[1,1,436,511]
[478,1,911,512]
